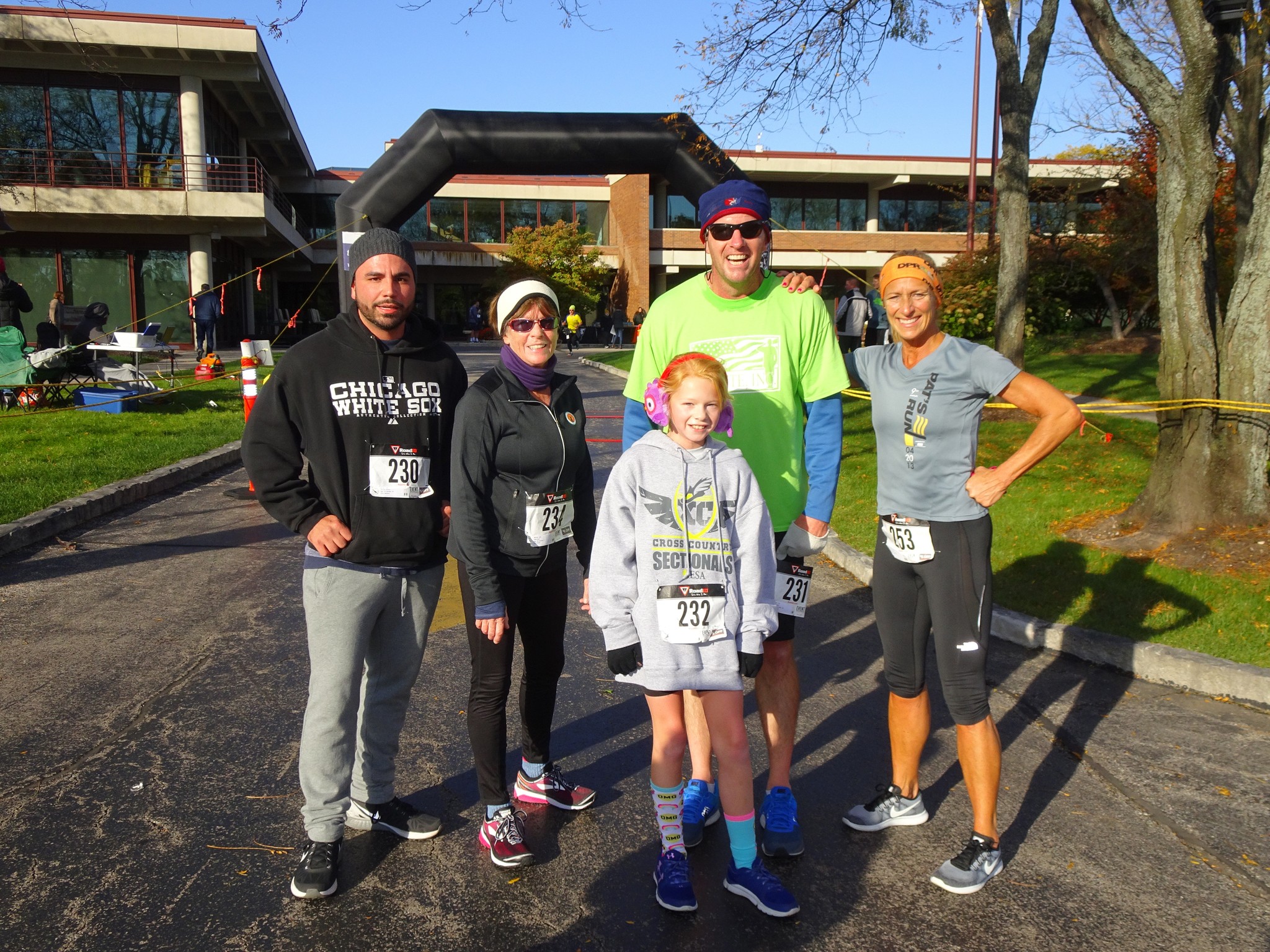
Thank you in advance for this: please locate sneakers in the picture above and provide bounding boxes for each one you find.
[842,781,929,832]
[514,763,603,810]
[682,779,720,846]
[653,848,698,910]
[477,803,536,869]
[930,830,1003,893]
[759,787,807,856]
[723,856,800,918]
[342,792,445,840]
[290,834,342,899]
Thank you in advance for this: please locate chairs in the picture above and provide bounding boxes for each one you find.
[0,322,99,413]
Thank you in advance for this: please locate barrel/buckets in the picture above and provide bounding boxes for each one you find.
[17,381,44,406]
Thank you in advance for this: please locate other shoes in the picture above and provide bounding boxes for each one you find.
[568,352,573,356]
[605,345,622,349]
[576,341,579,350]
[197,349,203,362]
[475,341,480,343]
[470,340,475,343]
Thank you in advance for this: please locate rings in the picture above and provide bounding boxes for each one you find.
[792,271,796,276]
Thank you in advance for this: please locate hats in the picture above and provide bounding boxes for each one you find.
[699,181,772,246]
[0,258,6,272]
[350,226,418,290]
[569,305,575,312]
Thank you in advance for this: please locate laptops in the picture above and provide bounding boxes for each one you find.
[89,326,127,346]
[156,326,176,345]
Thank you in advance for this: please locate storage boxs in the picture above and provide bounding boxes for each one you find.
[114,331,156,348]
[73,386,139,413]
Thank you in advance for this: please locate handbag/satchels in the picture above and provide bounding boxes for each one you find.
[47,317,52,324]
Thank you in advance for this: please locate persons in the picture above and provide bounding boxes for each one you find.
[241,227,468,899]
[0,256,322,410]
[775,248,1084,896]
[446,279,595,869]
[407,298,648,356]
[622,180,852,863]
[589,351,800,918]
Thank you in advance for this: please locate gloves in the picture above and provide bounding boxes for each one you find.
[776,519,831,562]
[737,652,764,678]
[607,645,643,675]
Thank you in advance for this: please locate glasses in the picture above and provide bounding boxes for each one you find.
[708,220,771,242]
[508,317,560,332]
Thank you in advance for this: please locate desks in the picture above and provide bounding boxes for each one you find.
[87,344,179,390]
